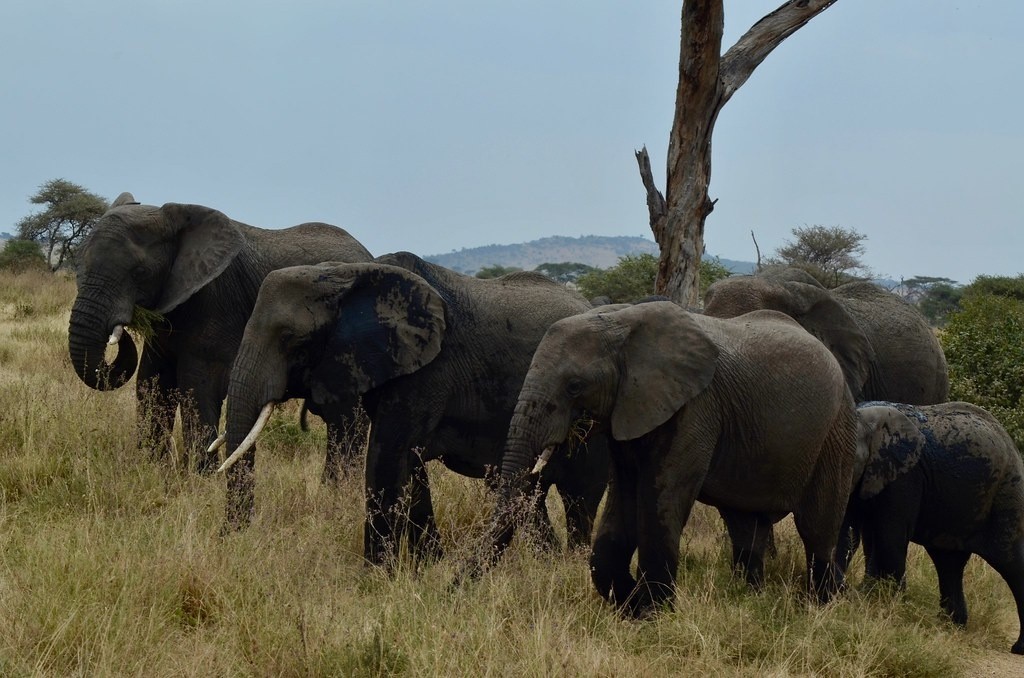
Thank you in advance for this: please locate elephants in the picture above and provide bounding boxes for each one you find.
[67,191,1024,656]
